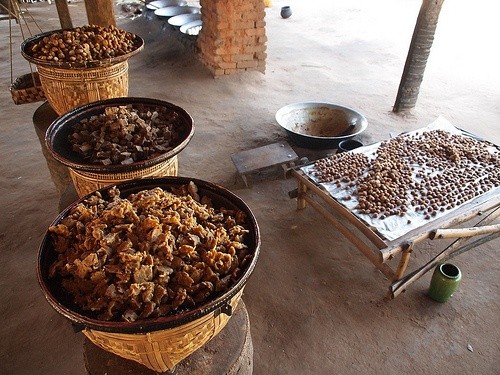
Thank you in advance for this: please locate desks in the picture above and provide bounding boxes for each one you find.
[286,122,500,293]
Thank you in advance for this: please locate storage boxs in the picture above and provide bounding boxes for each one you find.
[9,71,48,105]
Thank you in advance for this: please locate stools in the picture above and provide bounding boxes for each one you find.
[232,141,299,188]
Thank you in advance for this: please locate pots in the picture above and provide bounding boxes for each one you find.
[146,0,204,41]
[275,101,368,150]
